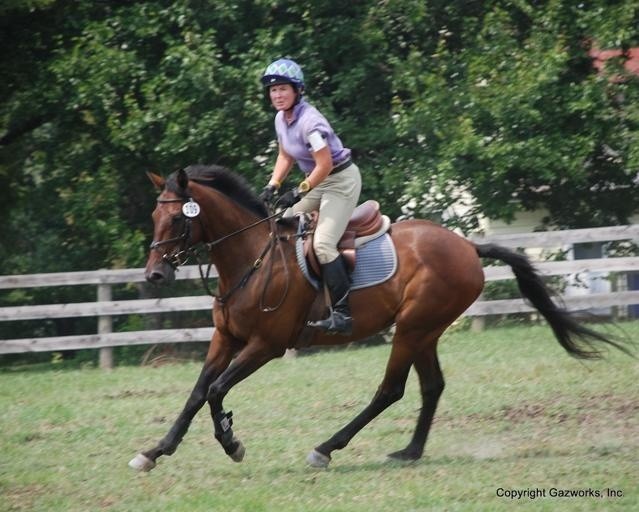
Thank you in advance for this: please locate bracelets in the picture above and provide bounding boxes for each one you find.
[298,181,311,196]
[267,180,281,190]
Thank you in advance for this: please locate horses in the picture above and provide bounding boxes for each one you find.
[127,163,638,472]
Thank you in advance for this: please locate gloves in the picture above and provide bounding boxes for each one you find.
[280,190,300,207]
[259,184,275,201]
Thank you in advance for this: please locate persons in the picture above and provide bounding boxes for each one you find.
[261,58,363,337]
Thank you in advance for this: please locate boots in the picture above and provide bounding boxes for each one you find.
[316,255,352,336]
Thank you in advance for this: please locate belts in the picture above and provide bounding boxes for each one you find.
[329,160,352,175]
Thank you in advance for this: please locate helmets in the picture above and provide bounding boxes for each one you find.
[260,60,305,91]
